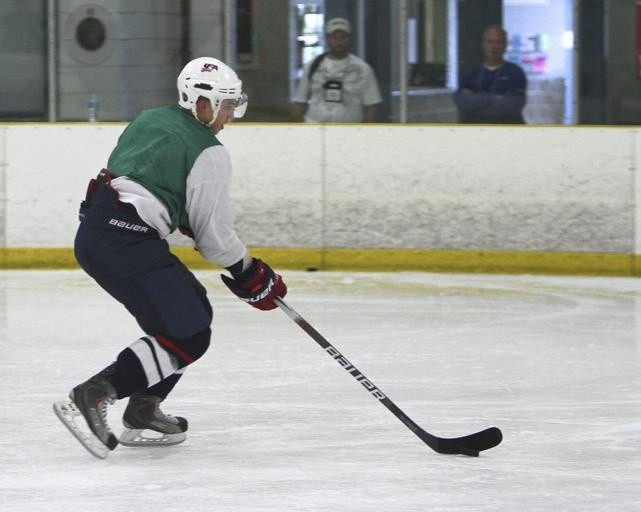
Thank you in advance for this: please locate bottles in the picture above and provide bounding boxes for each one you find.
[87,93,100,123]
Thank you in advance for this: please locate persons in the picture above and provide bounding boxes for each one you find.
[290,17,383,123]
[453,24,526,123]
[51,57,288,461]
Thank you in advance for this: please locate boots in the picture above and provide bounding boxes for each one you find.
[120,392,189,434]
[66,360,120,452]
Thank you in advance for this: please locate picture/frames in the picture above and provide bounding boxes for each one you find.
[224,0,259,74]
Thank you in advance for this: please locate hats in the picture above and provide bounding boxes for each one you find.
[324,16,352,36]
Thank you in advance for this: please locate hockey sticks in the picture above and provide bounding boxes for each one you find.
[274,294,503,455]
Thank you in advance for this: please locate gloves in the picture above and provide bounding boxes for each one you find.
[218,256,289,313]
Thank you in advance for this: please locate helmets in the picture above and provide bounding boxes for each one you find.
[172,55,248,120]
